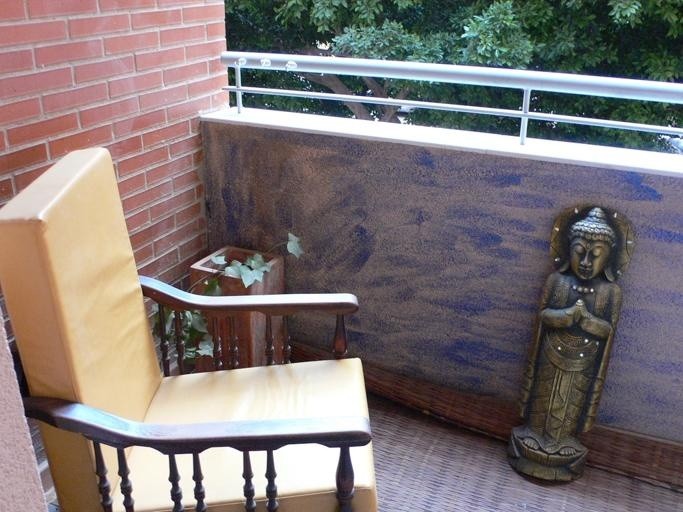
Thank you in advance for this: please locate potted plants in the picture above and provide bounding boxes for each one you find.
[151,231,306,374]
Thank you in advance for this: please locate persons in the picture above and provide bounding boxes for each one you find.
[518,205,636,456]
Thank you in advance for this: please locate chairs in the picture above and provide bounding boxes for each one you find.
[0,145,383,512]
